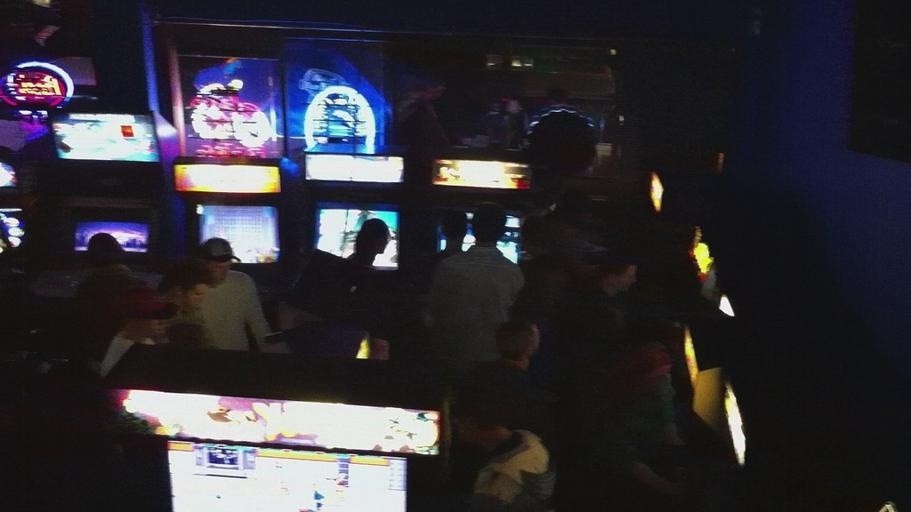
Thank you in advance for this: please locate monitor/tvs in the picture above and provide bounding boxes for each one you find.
[433,210,522,264]
[198,202,283,268]
[315,201,401,275]
[73,214,155,257]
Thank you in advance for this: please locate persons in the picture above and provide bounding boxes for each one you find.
[1,203,693,512]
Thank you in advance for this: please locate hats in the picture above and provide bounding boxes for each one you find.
[200,238,241,262]
